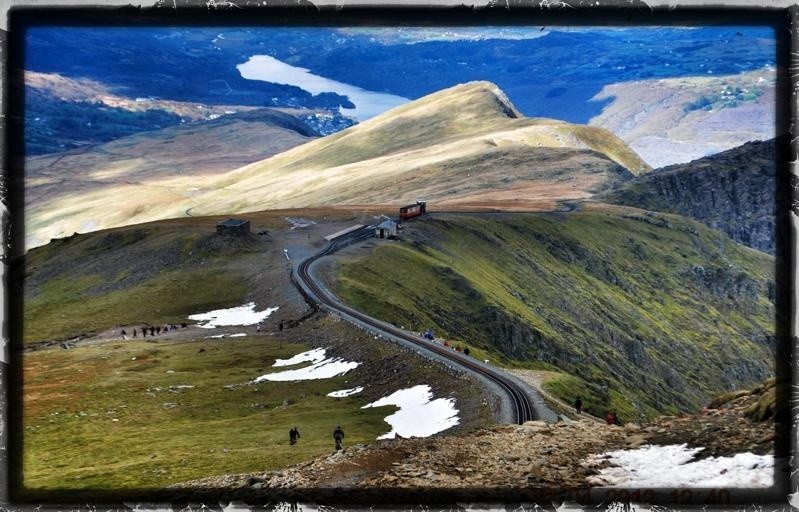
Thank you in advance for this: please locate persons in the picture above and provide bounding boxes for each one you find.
[575,396,582,413]
[121,326,160,339]
[290,427,300,440]
[608,407,621,426]
[334,426,344,448]
